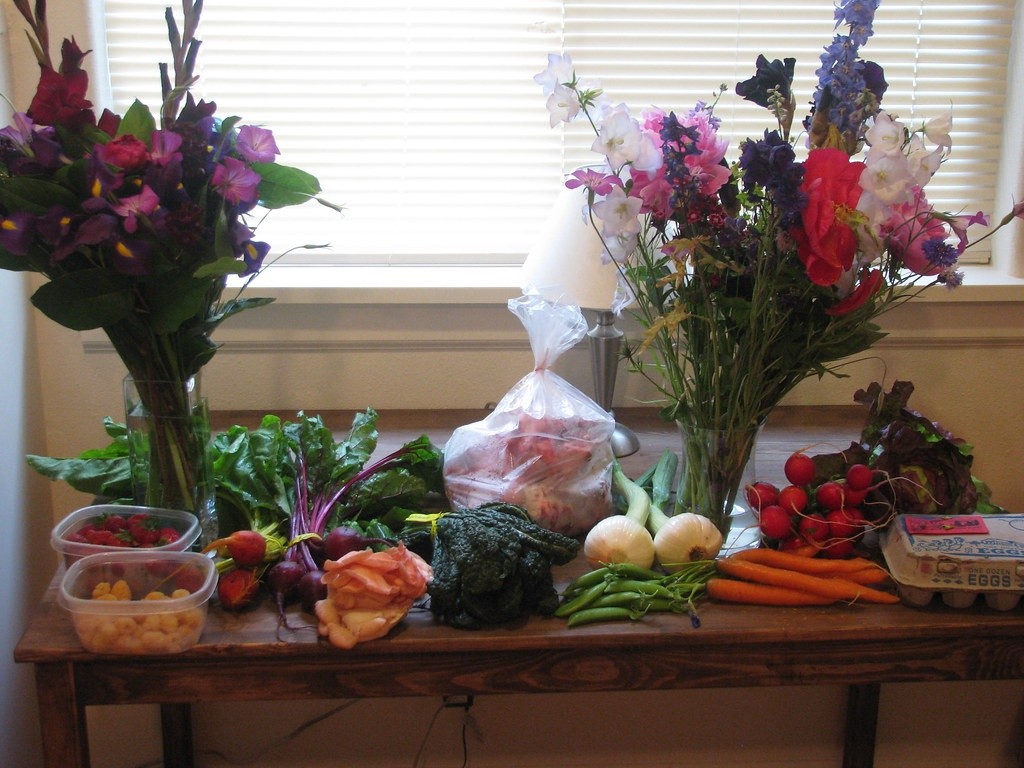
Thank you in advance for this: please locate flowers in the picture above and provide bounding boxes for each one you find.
[533,0,1024,544]
[0,0,349,552]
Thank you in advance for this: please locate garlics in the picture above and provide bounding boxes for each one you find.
[585,515,654,569]
[652,514,721,574]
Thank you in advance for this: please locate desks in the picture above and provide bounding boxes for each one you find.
[14,405,1024,768]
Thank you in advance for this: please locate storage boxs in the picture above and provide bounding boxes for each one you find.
[50,504,219,656]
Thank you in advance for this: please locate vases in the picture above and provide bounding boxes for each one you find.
[672,418,768,559]
[122,368,219,552]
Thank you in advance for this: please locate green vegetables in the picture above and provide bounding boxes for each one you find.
[26,410,578,633]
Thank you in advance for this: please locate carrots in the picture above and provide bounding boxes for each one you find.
[707,548,899,607]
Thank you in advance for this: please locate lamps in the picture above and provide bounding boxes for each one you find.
[518,163,694,458]
